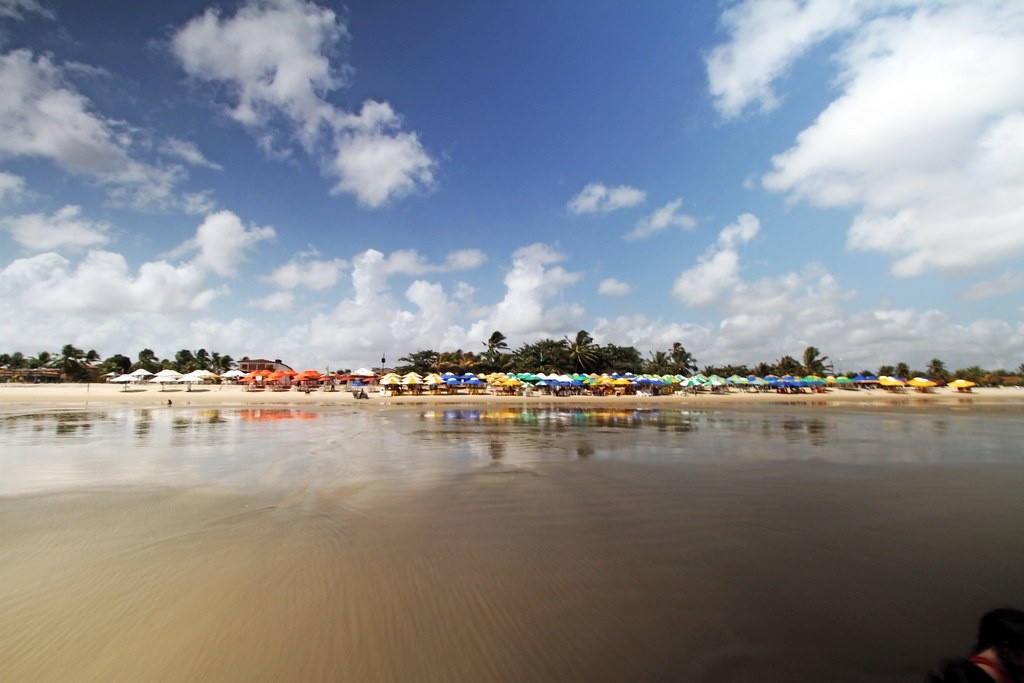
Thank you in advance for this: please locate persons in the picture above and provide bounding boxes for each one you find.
[927,608,1024,683]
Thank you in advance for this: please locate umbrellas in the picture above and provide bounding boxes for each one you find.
[107,369,975,395]
